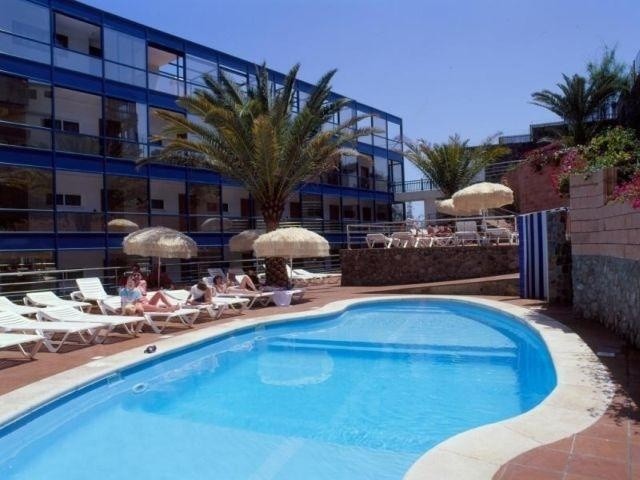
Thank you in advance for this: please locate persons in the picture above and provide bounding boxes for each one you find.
[130,268,181,314]
[118,275,146,334]
[212,274,265,295]
[183,279,216,306]
[245,268,286,292]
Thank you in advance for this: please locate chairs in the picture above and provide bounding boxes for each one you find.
[364,224,519,248]
[145,291,227,319]
[69,276,115,303]
[164,288,250,316]
[200,268,306,307]
[35,305,147,345]
[1,296,47,317]
[96,294,200,335]
[0,310,109,359]
[23,290,93,313]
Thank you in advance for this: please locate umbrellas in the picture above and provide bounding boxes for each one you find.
[253,227,331,288]
[106,217,139,232]
[122,225,200,291]
[199,217,236,232]
[452,181,515,238]
[436,198,484,241]
[227,228,263,274]
